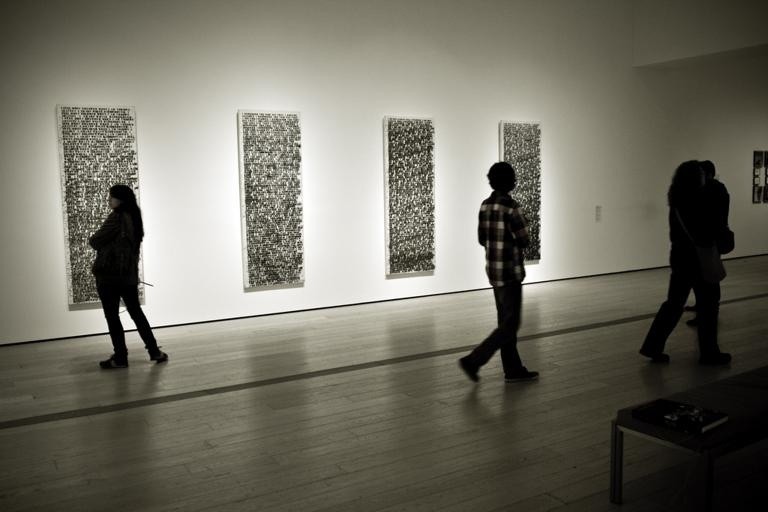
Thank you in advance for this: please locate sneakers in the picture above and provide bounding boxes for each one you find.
[638,348,671,364]
[504,368,540,383]
[459,357,480,383]
[698,352,732,366]
[99,357,129,369]
[150,351,168,362]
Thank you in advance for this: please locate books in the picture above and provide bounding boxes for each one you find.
[632,398,730,433]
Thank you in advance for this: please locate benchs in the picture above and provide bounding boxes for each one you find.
[609,366,768,512]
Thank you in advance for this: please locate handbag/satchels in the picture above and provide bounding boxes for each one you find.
[695,246,726,284]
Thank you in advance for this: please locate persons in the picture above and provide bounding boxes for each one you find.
[459,161,540,382]
[639,159,733,367]
[87,185,168,369]
[679,160,735,327]
